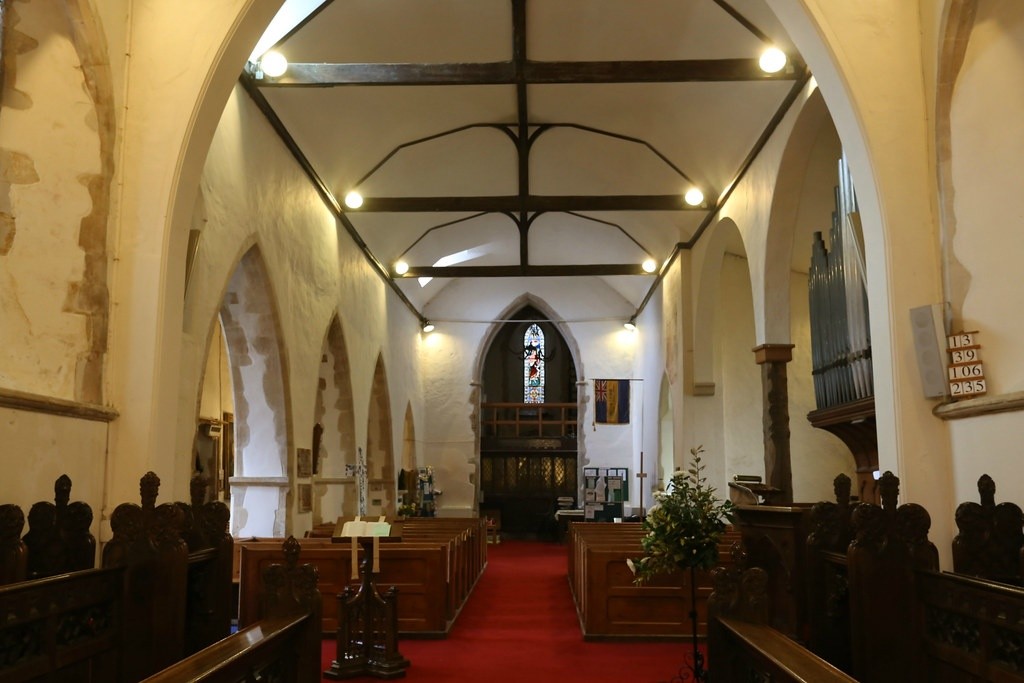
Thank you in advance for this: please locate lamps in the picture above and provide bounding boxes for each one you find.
[421,318,435,333]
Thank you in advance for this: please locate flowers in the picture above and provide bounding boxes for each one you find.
[626,444,738,588]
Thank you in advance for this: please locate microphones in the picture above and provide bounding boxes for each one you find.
[860,480,866,490]
[873,480,878,491]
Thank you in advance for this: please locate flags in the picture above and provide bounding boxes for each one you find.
[593,378,630,425]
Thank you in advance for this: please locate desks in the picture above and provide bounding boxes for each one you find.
[557,509,584,546]
[487,523,496,545]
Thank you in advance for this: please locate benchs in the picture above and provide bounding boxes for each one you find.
[233,518,487,638]
[567,522,746,642]
